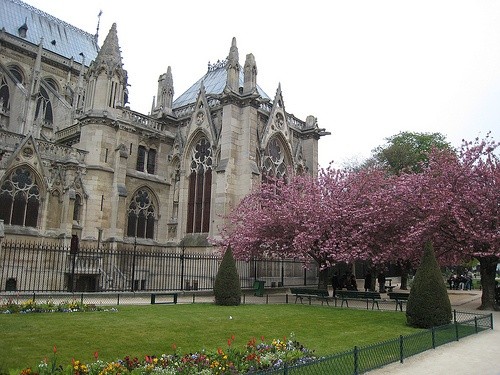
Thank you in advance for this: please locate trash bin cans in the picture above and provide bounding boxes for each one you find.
[253,280,266,298]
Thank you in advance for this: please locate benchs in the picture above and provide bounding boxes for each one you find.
[289,287,330,306]
[378,278,396,292]
[334,289,381,310]
[386,293,409,312]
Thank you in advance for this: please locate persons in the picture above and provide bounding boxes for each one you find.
[364,270,373,292]
[378,269,385,293]
[331,272,342,297]
[346,276,358,291]
[449,268,472,291]
